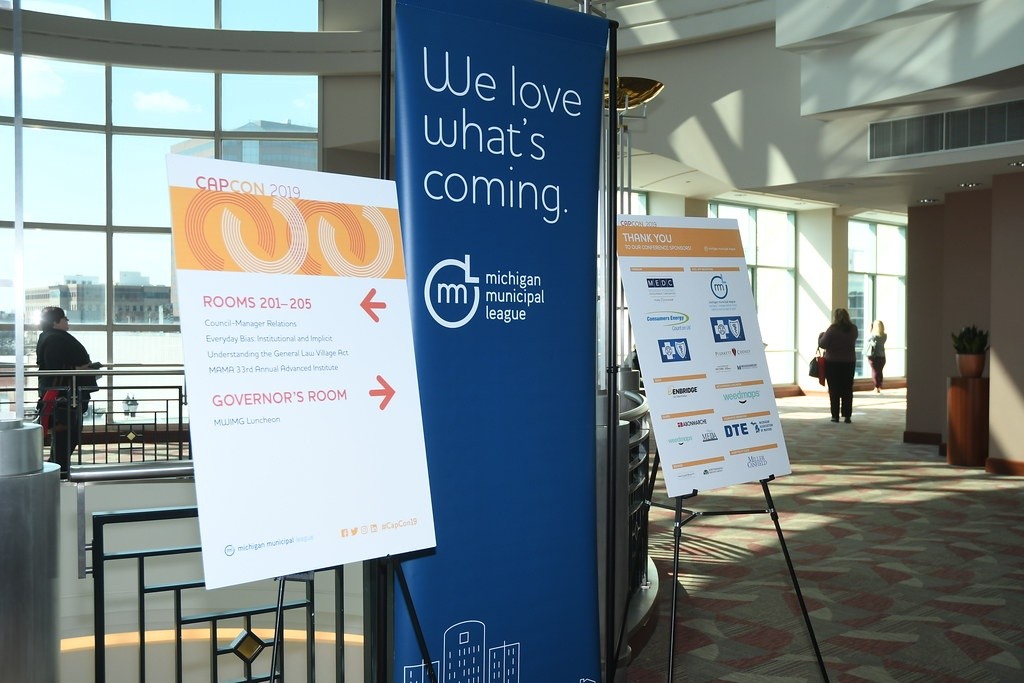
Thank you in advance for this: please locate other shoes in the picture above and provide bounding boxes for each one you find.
[831,417,852,423]
[874,387,880,393]
[59,469,68,480]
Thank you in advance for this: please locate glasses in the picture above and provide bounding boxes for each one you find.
[62,317,69,321]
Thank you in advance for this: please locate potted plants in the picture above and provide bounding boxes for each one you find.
[951,324,990,378]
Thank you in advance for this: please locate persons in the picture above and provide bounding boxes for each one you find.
[624,345,643,387]
[817,307,859,424]
[36,307,104,483]
[866,320,887,393]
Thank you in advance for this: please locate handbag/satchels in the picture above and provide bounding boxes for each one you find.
[862,342,875,356]
[809,345,826,386]
